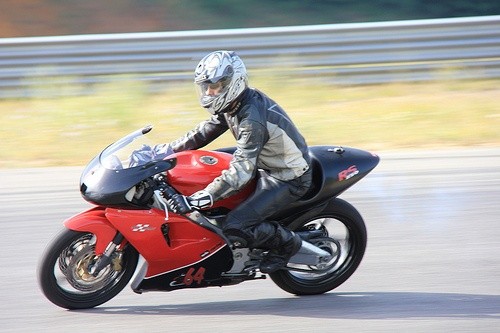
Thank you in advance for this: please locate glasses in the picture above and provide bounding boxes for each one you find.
[203,83,220,90]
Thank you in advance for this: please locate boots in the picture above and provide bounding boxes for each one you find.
[254,221,302,273]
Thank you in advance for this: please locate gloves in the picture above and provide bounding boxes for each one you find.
[129,142,173,168]
[166,190,213,215]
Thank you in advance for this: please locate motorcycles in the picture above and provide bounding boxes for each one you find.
[35,125,380,311]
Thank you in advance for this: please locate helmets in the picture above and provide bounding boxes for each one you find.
[194,51,248,115]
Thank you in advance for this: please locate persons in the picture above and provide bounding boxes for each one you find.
[141,50,313,274]
